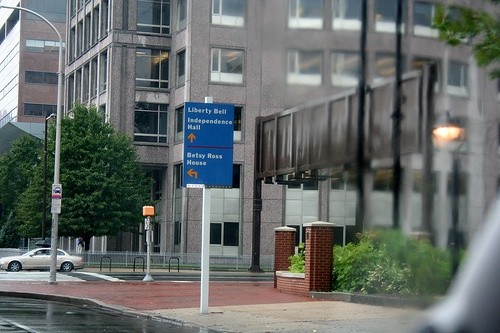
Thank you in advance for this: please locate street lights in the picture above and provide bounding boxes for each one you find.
[40,112,58,240]
[431,109,463,275]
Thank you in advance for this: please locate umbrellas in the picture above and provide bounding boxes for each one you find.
[34,239,51,246]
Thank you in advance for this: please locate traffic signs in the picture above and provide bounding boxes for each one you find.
[182,102,235,189]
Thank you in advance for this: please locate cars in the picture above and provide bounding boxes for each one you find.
[0,245,84,273]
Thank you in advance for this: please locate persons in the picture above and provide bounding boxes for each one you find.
[42,251,46,255]
[78,238,84,253]
